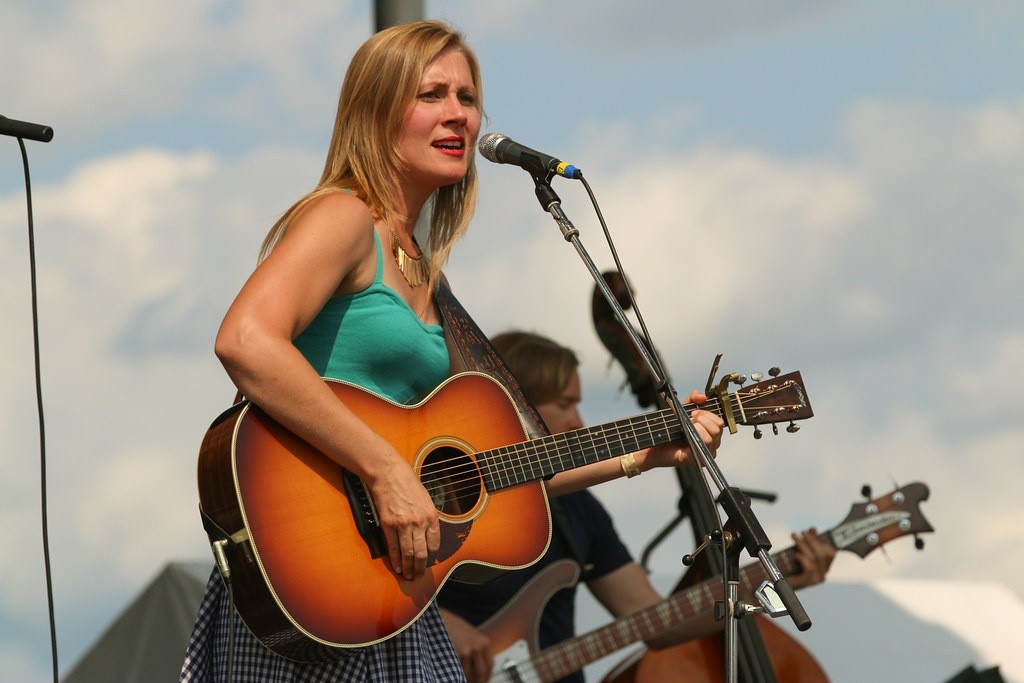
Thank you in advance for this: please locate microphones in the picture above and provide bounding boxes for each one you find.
[479,133,581,180]
[0,114,53,142]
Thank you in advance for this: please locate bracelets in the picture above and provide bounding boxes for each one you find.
[619,453,641,479]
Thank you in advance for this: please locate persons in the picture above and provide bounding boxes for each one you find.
[432,329,834,683]
[177,20,724,683]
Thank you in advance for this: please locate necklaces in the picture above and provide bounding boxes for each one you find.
[374,204,430,288]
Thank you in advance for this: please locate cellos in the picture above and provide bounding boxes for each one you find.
[590,266,834,683]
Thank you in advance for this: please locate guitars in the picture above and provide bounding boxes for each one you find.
[196,352,816,667]
[458,477,939,683]
